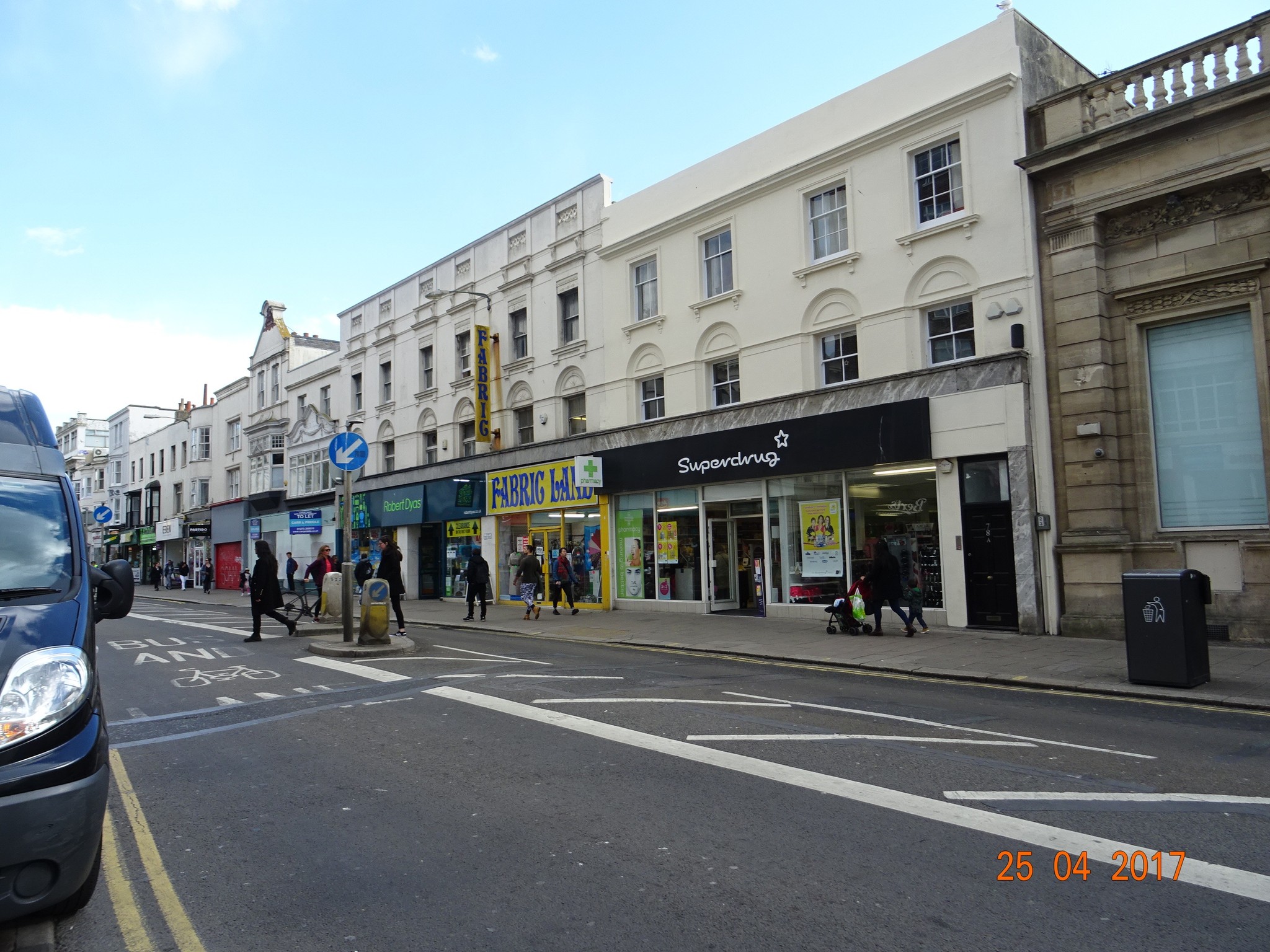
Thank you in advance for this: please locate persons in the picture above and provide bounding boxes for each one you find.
[862,529,879,557]
[806,517,816,542]
[376,535,407,636]
[714,544,730,599]
[625,539,641,566]
[90,540,375,642]
[463,547,489,621]
[901,579,929,633]
[513,545,543,620]
[625,568,642,596]
[508,551,522,595]
[824,516,835,540]
[860,540,917,637]
[814,515,825,535]
[552,547,581,615]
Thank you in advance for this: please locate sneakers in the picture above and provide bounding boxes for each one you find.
[481,617,486,621]
[463,617,474,621]
[389,630,407,636]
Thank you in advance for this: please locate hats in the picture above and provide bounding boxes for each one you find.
[245,567,249,570]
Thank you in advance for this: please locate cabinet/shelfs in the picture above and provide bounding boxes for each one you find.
[913,523,943,608]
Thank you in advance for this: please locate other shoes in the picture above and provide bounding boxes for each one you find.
[245,634,262,643]
[533,606,541,620]
[358,600,362,606]
[553,609,560,615]
[287,620,297,636]
[208,590,210,594]
[247,593,251,596]
[165,586,172,590]
[155,588,159,590]
[313,618,319,623]
[901,627,908,632]
[906,625,916,637]
[241,593,244,596]
[572,609,579,615]
[204,591,206,593]
[868,628,883,636]
[921,628,930,633]
[523,614,530,620]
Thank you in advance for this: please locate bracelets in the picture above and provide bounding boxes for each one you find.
[513,579,517,582]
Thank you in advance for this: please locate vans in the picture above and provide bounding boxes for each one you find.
[0,384,137,928]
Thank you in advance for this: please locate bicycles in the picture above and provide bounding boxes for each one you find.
[275,579,322,623]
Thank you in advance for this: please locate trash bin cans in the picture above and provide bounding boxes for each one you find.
[1122,568,1212,688]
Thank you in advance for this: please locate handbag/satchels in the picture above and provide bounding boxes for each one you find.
[849,587,866,622]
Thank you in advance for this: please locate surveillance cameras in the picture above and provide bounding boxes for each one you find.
[1094,448,1105,457]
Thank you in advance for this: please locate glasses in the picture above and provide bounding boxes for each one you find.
[205,560,209,561]
[324,549,331,551]
